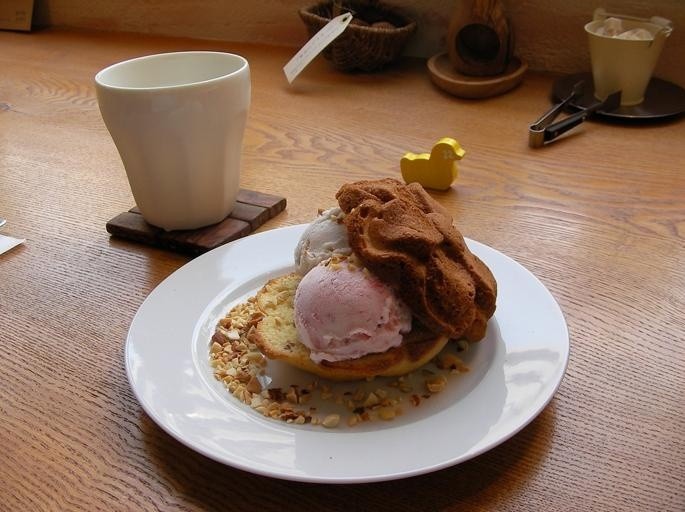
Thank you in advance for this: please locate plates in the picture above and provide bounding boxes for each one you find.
[550,72,685,123]
[122,222,571,486]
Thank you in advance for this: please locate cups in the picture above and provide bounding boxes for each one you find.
[584,7,672,107]
[93,51,252,231]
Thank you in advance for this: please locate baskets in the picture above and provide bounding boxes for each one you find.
[295,0,421,73]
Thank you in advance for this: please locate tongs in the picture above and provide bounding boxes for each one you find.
[528,76,622,148]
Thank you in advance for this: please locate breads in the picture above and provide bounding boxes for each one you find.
[252,269,449,385]
